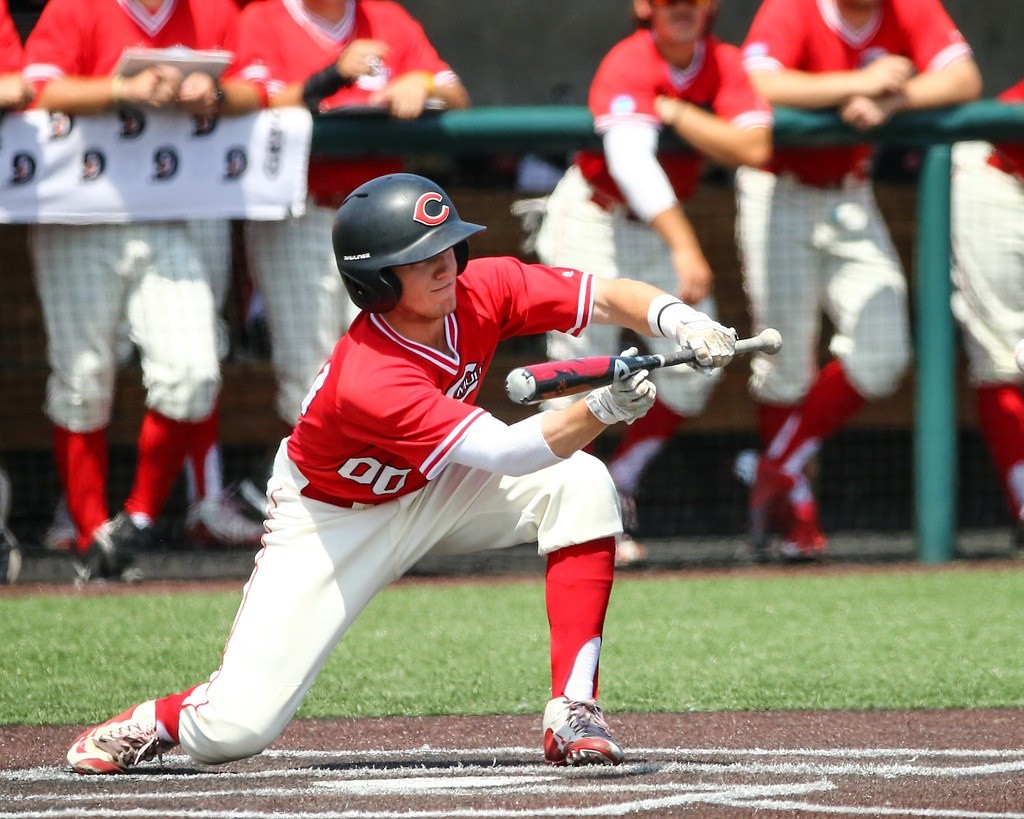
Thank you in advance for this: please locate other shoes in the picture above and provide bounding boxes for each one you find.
[74,508,161,582]
[614,533,651,568]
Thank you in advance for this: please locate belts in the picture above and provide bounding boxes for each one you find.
[591,191,646,223]
[299,482,355,508]
[759,163,839,190]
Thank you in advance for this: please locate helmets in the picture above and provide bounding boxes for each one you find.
[332,173,487,313]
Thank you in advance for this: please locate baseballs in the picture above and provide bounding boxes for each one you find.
[832,200,871,232]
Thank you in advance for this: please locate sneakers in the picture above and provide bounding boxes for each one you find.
[181,483,267,552]
[44,494,77,550]
[542,696,625,767]
[66,699,177,775]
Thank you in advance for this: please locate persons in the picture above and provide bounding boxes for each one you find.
[536,0,775,574]
[0,0,25,111]
[742,0,982,567]
[59,171,736,776]
[949,70,1024,561]
[239,1,471,523]
[24,0,270,618]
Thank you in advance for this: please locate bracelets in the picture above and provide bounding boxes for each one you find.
[425,71,434,98]
[110,72,124,110]
[210,68,225,113]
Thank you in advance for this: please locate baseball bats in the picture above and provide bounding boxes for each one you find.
[506,327,783,406]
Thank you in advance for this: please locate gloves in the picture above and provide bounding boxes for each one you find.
[584,347,656,425]
[676,312,739,378]
